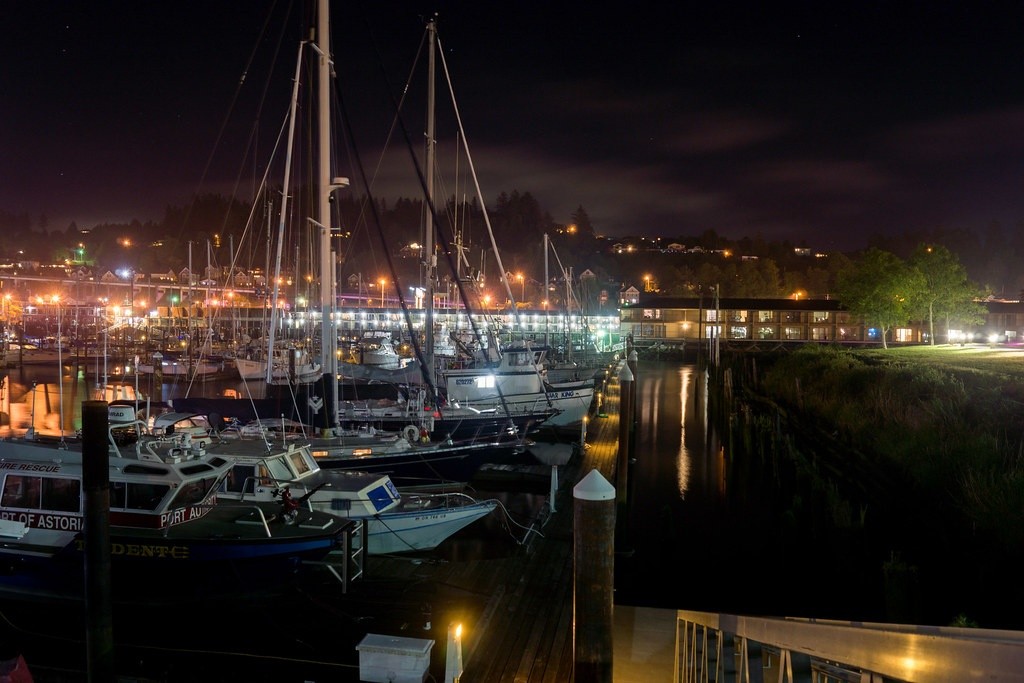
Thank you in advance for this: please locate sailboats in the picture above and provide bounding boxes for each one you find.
[0,2,609,607]
